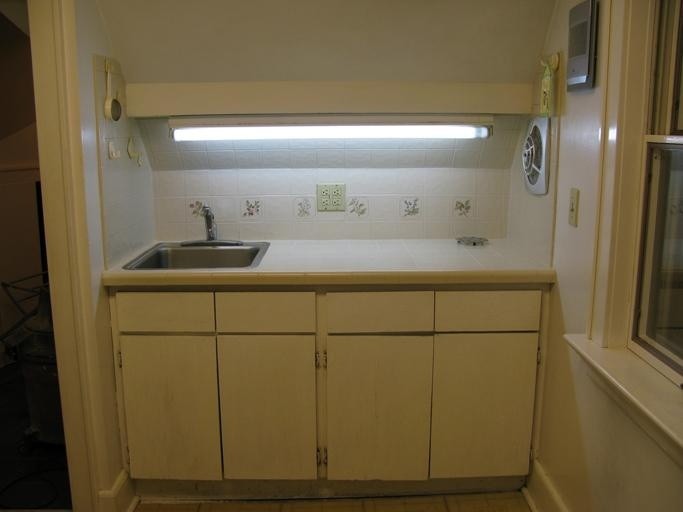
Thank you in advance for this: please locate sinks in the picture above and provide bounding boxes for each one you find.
[122,242,272,270]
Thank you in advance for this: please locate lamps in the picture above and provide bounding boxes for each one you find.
[166,114,495,146]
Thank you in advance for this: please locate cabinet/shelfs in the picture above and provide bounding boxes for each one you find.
[107,287,321,482]
[317,287,545,482]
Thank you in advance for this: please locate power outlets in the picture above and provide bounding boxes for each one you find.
[317,183,346,212]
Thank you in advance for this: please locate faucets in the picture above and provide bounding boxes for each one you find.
[200,205,216,240]
